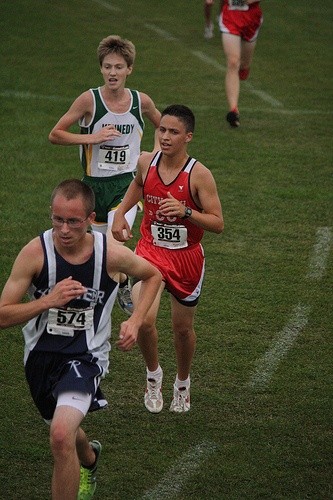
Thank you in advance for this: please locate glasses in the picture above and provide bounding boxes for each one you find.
[49,209,90,228]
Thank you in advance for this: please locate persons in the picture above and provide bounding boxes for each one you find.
[0,179,163,500]
[49,35,161,315]
[203,0,224,41]
[111,104,224,413]
[218,0,264,127]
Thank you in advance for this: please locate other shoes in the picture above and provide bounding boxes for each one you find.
[227,109,241,127]
[204,25,212,39]
[240,67,250,80]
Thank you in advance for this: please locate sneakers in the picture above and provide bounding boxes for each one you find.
[169,382,190,413]
[145,379,163,413]
[77,440,102,500]
[116,278,135,316]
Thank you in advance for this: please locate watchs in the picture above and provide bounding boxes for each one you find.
[182,207,192,220]
